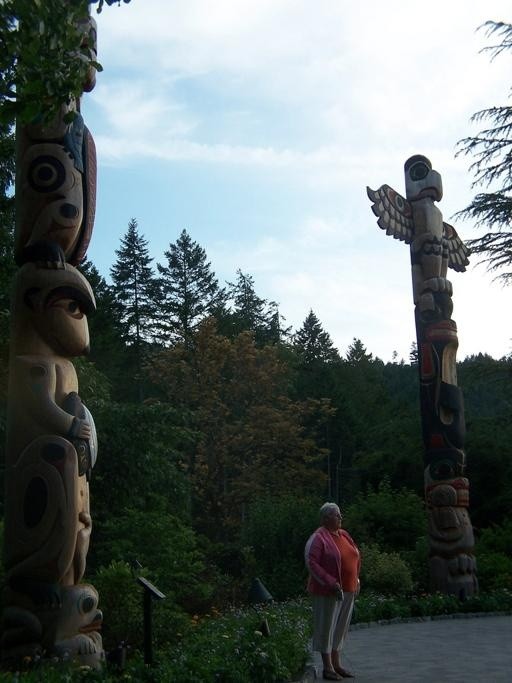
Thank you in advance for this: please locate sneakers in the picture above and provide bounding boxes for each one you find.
[323,668,355,681]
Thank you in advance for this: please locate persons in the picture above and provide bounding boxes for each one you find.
[304,500,362,681]
